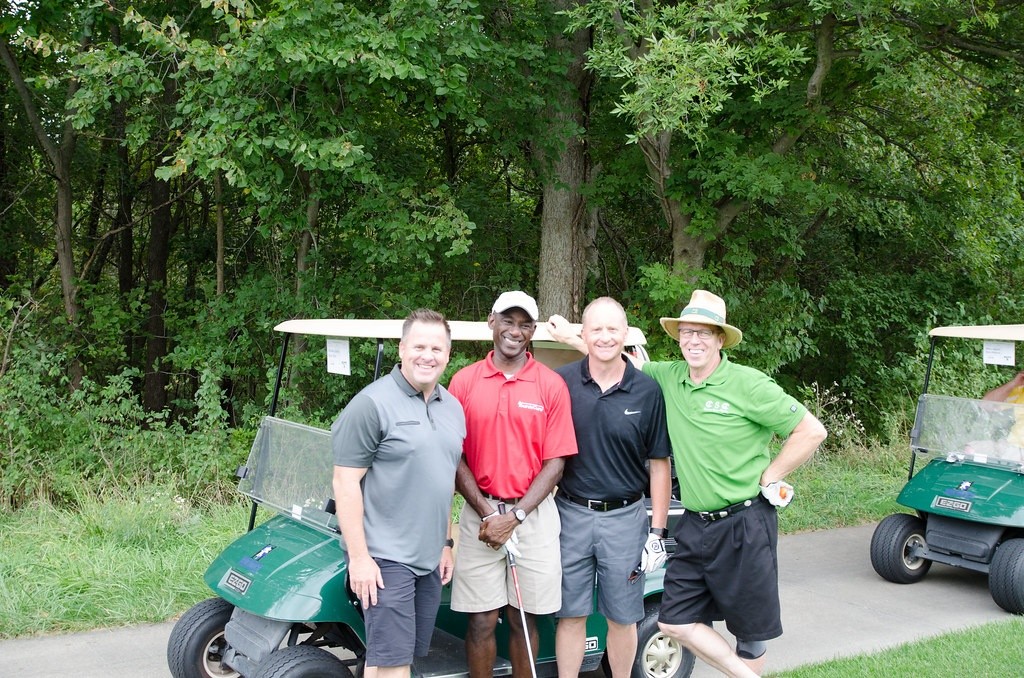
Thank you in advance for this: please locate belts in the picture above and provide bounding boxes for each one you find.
[481,491,522,505]
[557,485,643,511]
[686,496,767,523]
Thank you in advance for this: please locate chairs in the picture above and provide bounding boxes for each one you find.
[965,440,1024,461]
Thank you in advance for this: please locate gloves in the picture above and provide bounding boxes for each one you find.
[759,480,795,507]
[641,532,667,575]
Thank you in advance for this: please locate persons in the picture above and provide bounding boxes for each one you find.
[982,371,1024,462]
[331,311,466,678]
[553,297,672,678]
[547,290,827,678]
[448,290,577,678]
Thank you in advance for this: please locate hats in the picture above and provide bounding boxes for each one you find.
[659,290,743,348]
[492,291,539,320]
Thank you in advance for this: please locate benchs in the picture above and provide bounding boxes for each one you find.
[980,372,1024,403]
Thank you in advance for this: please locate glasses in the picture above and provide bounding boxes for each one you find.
[628,561,645,585]
[677,328,721,339]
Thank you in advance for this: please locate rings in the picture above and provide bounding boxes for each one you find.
[487,543,490,547]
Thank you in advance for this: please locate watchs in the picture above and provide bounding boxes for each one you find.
[650,528,669,538]
[511,507,526,524]
[445,538,454,548]
